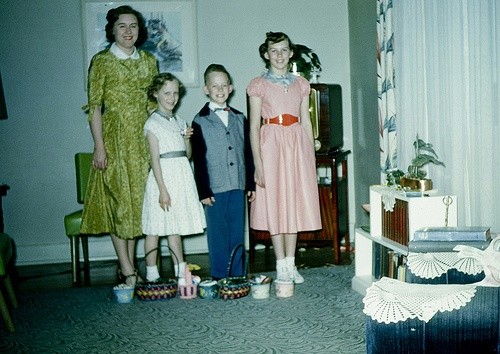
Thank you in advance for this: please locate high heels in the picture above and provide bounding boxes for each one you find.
[119,265,143,286]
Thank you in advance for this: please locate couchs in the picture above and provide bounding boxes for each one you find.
[362,233,500,354]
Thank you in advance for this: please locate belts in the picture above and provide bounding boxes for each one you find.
[265,113,299,127]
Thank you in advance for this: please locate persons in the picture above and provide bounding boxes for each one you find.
[247,32,322,283]
[80,6,159,288]
[142,72,208,286]
[190,63,256,283]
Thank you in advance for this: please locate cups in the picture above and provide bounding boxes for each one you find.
[274,279,295,297]
[113,287,133,304]
[250,283,270,299]
[199,280,218,299]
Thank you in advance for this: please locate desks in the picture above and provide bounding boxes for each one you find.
[247,150,350,264]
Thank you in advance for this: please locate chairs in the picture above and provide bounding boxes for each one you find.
[64,152,96,282]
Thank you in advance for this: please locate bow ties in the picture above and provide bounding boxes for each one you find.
[214,107,230,113]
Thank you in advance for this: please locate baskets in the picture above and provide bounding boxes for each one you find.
[135,245,180,300]
[217,244,250,300]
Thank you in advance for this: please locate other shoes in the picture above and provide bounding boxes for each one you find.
[277,264,304,283]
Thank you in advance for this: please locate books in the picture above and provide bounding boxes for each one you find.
[413,226,491,241]
